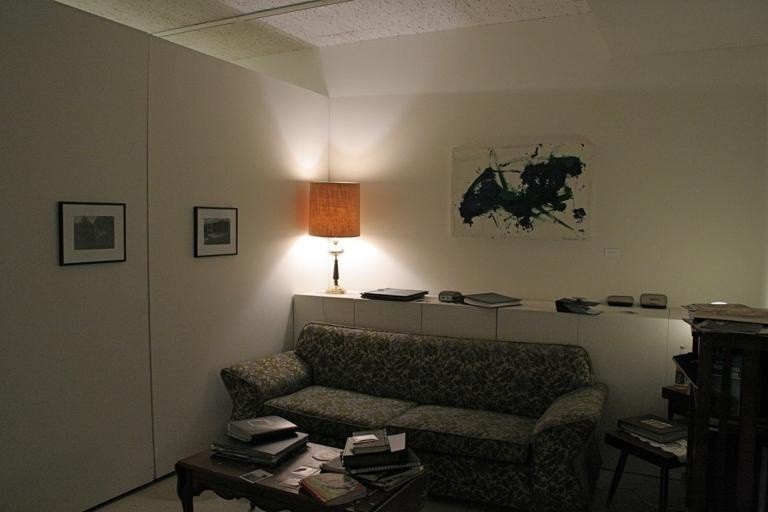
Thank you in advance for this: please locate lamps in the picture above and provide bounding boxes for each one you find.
[307,180,362,292]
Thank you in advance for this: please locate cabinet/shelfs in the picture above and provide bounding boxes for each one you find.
[672,315,768,511]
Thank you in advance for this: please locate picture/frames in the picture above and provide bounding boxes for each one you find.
[192,205,238,258]
[56,200,128,267]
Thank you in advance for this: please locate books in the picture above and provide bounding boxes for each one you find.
[226,415,298,443]
[555,298,603,315]
[209,432,310,467]
[319,428,426,491]
[362,289,429,302]
[461,292,522,307]
[681,303,768,324]
[617,415,688,443]
[298,473,367,507]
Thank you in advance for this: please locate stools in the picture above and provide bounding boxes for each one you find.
[605,425,712,511]
[660,385,690,420]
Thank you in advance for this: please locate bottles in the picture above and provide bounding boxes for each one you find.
[674,344,688,389]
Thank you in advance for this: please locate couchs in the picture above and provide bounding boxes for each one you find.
[218,322,609,510]
